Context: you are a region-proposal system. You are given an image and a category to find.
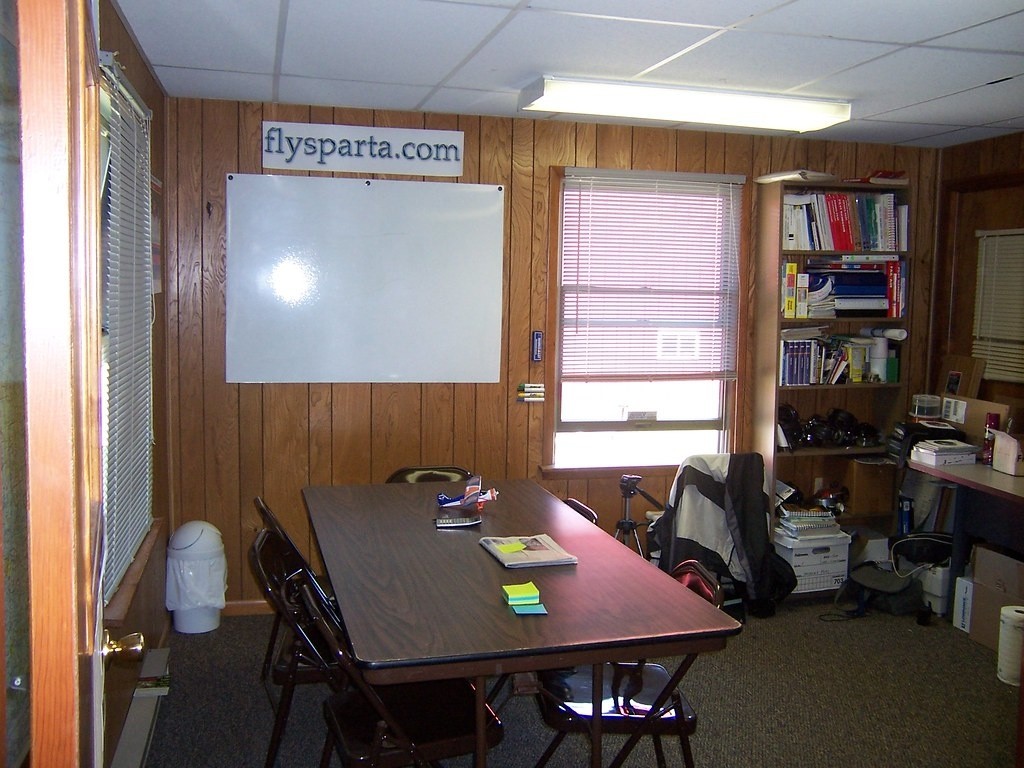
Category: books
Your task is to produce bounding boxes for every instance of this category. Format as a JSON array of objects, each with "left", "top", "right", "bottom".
[
  {"left": 915, "top": 441, "right": 979, "bottom": 467},
  {"left": 781, "top": 191, "right": 907, "bottom": 385},
  {"left": 773, "top": 501, "right": 840, "bottom": 539}
]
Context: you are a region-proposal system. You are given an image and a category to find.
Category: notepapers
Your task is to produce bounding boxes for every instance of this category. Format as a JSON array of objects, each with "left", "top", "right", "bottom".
[{"left": 502, "top": 581, "right": 540, "bottom": 605}]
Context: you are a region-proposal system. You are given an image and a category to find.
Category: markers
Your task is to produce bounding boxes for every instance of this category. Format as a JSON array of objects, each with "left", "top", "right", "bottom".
[{"left": 517, "top": 384, "right": 545, "bottom": 402}]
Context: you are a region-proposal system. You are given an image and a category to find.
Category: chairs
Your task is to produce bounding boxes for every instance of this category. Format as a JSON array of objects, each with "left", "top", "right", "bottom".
[{"left": 246, "top": 466, "right": 724, "bottom": 767}]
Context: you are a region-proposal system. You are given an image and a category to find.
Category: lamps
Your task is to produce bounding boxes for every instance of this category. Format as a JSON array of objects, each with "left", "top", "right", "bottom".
[{"left": 516, "top": 75, "right": 852, "bottom": 134}]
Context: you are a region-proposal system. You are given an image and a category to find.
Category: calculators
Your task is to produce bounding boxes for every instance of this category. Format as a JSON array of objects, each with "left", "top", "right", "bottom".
[{"left": 436, "top": 515, "right": 482, "bottom": 528}]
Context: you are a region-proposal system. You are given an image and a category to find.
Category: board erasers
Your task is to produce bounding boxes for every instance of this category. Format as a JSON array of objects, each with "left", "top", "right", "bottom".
[{"left": 533, "top": 331, "right": 543, "bottom": 361}]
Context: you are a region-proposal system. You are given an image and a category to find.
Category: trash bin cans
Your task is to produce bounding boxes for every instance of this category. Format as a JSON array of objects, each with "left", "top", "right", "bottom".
[{"left": 167, "top": 520, "right": 225, "bottom": 634}]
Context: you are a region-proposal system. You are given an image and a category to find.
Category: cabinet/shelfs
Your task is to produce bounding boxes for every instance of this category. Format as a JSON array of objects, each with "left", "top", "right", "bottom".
[{"left": 755, "top": 181, "right": 915, "bottom": 546}]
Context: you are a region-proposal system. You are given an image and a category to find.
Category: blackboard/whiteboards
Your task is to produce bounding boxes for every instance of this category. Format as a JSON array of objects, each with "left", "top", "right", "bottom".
[{"left": 226, "top": 173, "right": 504, "bottom": 383}]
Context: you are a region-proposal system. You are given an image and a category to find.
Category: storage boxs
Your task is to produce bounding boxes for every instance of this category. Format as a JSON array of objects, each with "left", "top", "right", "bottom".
[
  {"left": 965, "top": 544, "right": 1024, "bottom": 653},
  {"left": 784, "top": 263, "right": 797, "bottom": 318},
  {"left": 950, "top": 578, "right": 975, "bottom": 634},
  {"left": 831, "top": 456, "right": 893, "bottom": 517},
  {"left": 773, "top": 534, "right": 849, "bottom": 594},
  {"left": 934, "top": 354, "right": 1024, "bottom": 476},
  {"left": 841, "top": 343, "right": 862, "bottom": 383},
  {"left": 796, "top": 274, "right": 809, "bottom": 319},
  {"left": 894, "top": 555, "right": 952, "bottom": 619}
]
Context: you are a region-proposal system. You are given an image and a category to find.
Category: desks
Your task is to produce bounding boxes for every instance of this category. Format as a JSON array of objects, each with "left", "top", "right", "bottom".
[
  {"left": 905, "top": 457, "right": 1024, "bottom": 537},
  {"left": 299, "top": 484, "right": 743, "bottom": 767}
]
